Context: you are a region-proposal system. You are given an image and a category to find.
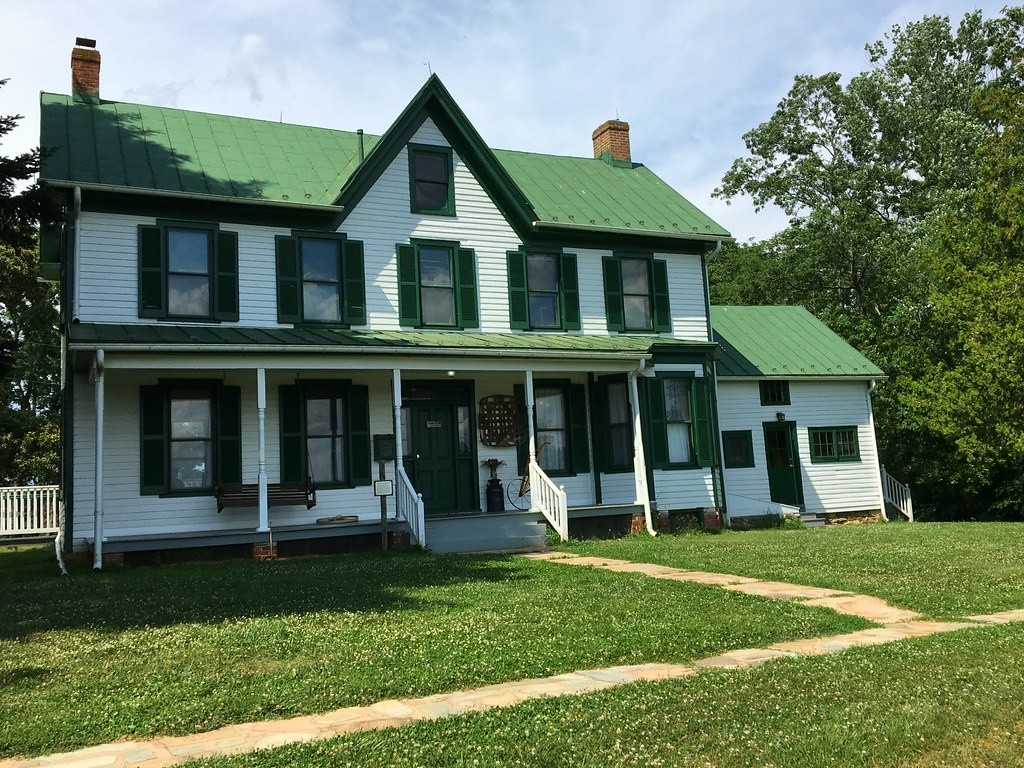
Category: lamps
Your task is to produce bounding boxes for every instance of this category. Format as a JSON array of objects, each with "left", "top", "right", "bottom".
[{"left": 776, "top": 411, "right": 786, "bottom": 421}]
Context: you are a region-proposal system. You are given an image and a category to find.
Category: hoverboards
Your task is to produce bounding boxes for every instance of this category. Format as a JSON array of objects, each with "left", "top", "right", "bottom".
[{"left": 506, "top": 441, "right": 552, "bottom": 511}]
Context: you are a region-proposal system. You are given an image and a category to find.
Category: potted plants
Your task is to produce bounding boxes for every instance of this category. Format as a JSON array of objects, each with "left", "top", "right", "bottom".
[{"left": 482, "top": 457, "right": 508, "bottom": 513}]
